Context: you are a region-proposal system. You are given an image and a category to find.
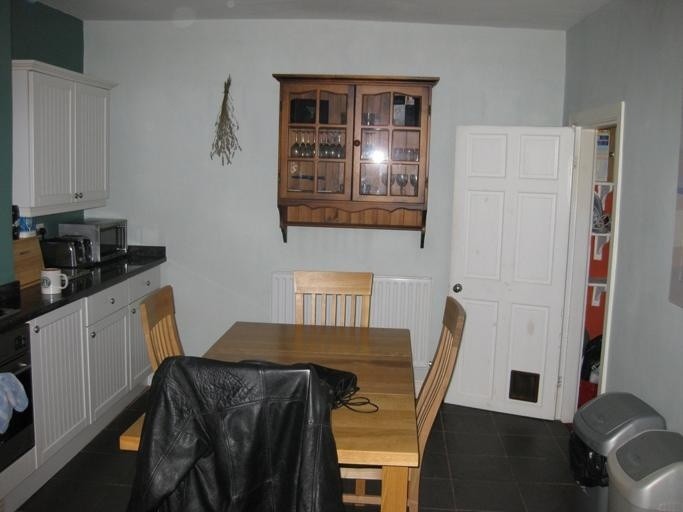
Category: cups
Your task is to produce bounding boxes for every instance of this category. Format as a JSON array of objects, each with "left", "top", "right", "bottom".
[
  {"left": 364, "top": 112, "right": 375, "bottom": 125},
  {"left": 324, "top": 130, "right": 330, "bottom": 158},
  {"left": 330, "top": 130, "right": 337, "bottom": 158},
  {"left": 40, "top": 268, "right": 68, "bottom": 294},
  {"left": 397, "top": 174, "right": 408, "bottom": 196},
  {"left": 311, "top": 130, "right": 315, "bottom": 152},
  {"left": 410, "top": 175, "right": 418, "bottom": 195},
  {"left": 319, "top": 129, "right": 324, "bottom": 158},
  {"left": 383, "top": 173, "right": 395, "bottom": 194},
  {"left": 300, "top": 130, "right": 306, "bottom": 148},
  {"left": 337, "top": 130, "right": 344, "bottom": 158},
  {"left": 303, "top": 130, "right": 313, "bottom": 159},
  {"left": 291, "top": 130, "right": 300, "bottom": 157}
]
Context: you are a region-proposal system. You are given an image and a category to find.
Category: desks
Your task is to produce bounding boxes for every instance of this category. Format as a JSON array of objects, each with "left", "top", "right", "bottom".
[{"left": 120, "top": 321, "right": 419, "bottom": 511}]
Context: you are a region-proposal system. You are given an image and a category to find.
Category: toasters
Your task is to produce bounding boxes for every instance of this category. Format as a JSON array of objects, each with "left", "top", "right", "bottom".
[{"left": 40, "top": 235, "right": 92, "bottom": 270}]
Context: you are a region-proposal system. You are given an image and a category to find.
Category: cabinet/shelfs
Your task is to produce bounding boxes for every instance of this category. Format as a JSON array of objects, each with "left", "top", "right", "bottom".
[
  {"left": 27, "top": 300, "right": 90, "bottom": 469},
  {"left": 11, "top": 59, "right": 119, "bottom": 217},
  {"left": 272, "top": 73, "right": 440, "bottom": 249},
  {"left": 85, "top": 266, "right": 161, "bottom": 424}
]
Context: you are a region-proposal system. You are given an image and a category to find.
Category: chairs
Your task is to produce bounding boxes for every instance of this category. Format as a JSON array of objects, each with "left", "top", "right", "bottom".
[
  {"left": 294, "top": 271, "right": 373, "bottom": 327},
  {"left": 340, "top": 297, "right": 466, "bottom": 512},
  {"left": 140, "top": 285, "right": 185, "bottom": 372}
]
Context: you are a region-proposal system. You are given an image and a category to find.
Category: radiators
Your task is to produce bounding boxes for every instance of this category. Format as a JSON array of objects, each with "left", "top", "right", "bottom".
[{"left": 272, "top": 270, "right": 432, "bottom": 368}]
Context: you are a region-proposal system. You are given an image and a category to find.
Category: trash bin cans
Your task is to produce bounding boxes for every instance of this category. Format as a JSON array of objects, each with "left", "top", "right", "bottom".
[
  {"left": 572, "top": 391, "right": 667, "bottom": 511},
  {"left": 604, "top": 427, "right": 683, "bottom": 512}
]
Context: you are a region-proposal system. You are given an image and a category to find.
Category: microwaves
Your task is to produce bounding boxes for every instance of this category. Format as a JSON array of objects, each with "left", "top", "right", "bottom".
[{"left": 59, "top": 217, "right": 127, "bottom": 262}]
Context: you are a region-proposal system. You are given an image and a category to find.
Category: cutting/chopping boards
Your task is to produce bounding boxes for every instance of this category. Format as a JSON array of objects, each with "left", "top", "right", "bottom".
[{"left": 13, "top": 236, "right": 44, "bottom": 289}]
